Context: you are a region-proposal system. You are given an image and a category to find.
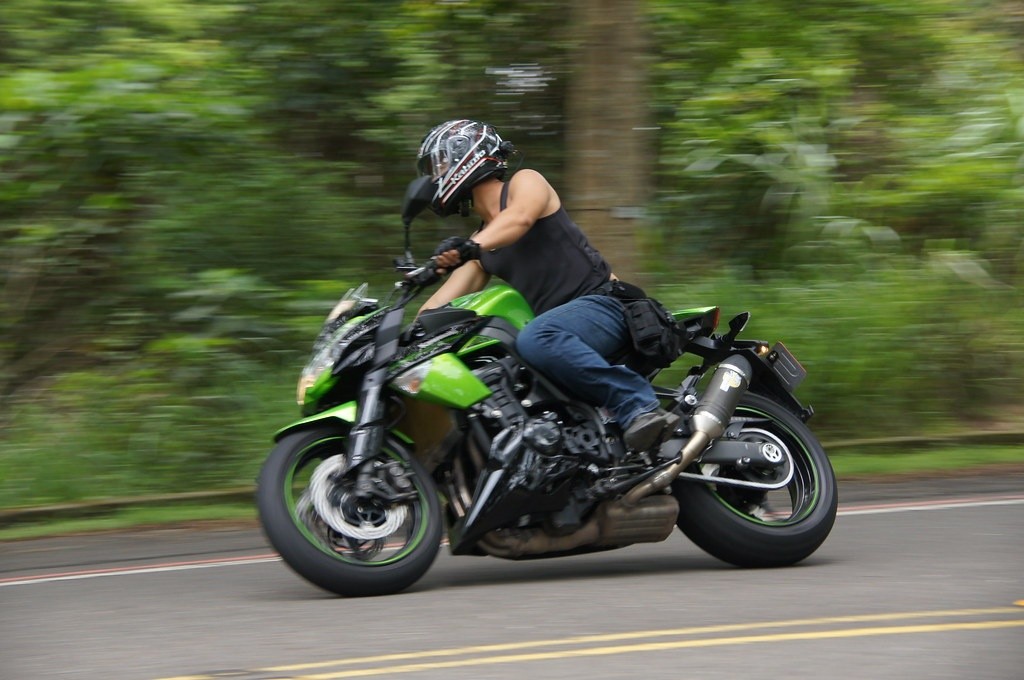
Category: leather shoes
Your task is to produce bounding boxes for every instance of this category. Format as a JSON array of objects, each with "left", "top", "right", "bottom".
[{"left": 625, "top": 407, "right": 681, "bottom": 451}]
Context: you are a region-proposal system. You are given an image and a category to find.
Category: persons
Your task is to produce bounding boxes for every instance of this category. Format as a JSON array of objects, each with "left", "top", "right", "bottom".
[{"left": 414, "top": 119, "right": 679, "bottom": 450}]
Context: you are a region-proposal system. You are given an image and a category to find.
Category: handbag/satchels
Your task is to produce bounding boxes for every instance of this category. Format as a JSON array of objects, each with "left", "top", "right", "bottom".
[{"left": 626, "top": 297, "right": 678, "bottom": 360}]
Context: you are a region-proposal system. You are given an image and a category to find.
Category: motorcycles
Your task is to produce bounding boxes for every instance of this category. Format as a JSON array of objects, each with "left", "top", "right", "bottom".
[{"left": 253, "top": 176, "right": 839, "bottom": 599}]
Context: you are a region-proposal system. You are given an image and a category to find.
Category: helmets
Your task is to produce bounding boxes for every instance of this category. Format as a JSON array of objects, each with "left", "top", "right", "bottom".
[{"left": 417, "top": 118, "right": 510, "bottom": 216}]
